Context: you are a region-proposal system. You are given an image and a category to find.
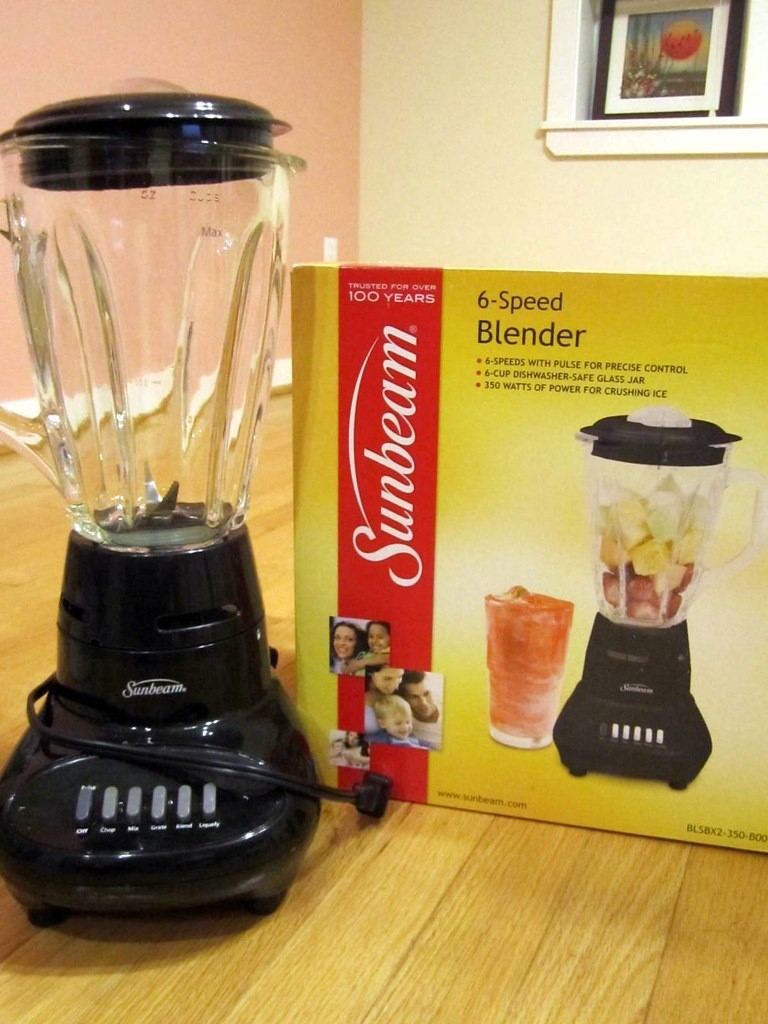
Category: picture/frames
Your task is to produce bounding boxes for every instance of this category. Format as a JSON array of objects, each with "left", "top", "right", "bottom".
[{"left": 592, "top": 0, "right": 744, "bottom": 119}]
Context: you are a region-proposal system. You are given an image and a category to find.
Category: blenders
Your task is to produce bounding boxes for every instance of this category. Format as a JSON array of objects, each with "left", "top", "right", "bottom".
[
  {"left": 548, "top": 405, "right": 747, "bottom": 793},
  {"left": 0, "top": 69, "right": 323, "bottom": 926}
]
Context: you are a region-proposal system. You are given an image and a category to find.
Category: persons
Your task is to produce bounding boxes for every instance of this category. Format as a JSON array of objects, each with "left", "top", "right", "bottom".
[
  {"left": 398, "top": 668, "right": 444, "bottom": 748},
  {"left": 328, "top": 621, "right": 365, "bottom": 676},
  {"left": 365, "top": 695, "right": 436, "bottom": 749},
  {"left": 339, "top": 621, "right": 391, "bottom": 677},
  {"left": 329, "top": 729, "right": 372, "bottom": 771},
  {"left": 365, "top": 662, "right": 404, "bottom": 734}
]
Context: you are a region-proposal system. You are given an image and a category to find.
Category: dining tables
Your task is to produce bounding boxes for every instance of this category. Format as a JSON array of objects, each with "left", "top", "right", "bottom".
[{"left": 0, "top": 386, "right": 768, "bottom": 1024}]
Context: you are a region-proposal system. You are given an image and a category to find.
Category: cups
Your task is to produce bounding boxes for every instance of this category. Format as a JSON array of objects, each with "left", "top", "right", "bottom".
[{"left": 482, "top": 594, "right": 573, "bottom": 753}]
